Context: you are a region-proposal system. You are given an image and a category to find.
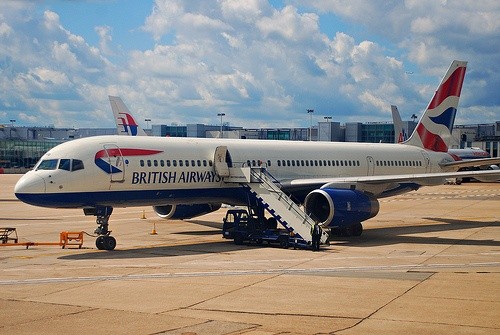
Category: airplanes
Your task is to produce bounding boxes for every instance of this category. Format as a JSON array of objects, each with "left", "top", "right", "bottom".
[{"left": 14, "top": 59, "right": 500, "bottom": 252}]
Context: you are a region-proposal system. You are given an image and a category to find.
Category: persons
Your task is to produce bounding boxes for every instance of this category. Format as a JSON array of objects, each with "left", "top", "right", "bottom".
[{"left": 310, "top": 221, "right": 322, "bottom": 252}]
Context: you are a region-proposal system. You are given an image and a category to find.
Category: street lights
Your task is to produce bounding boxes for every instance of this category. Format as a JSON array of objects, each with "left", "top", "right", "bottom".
[
  {"left": 411, "top": 114, "right": 418, "bottom": 122},
  {"left": 307, "top": 108, "right": 314, "bottom": 141},
  {"left": 217, "top": 113, "right": 225, "bottom": 133},
  {"left": 324, "top": 116, "right": 333, "bottom": 122},
  {"left": 145, "top": 118, "right": 151, "bottom": 128},
  {"left": 10, "top": 119, "right": 16, "bottom": 125}
]
